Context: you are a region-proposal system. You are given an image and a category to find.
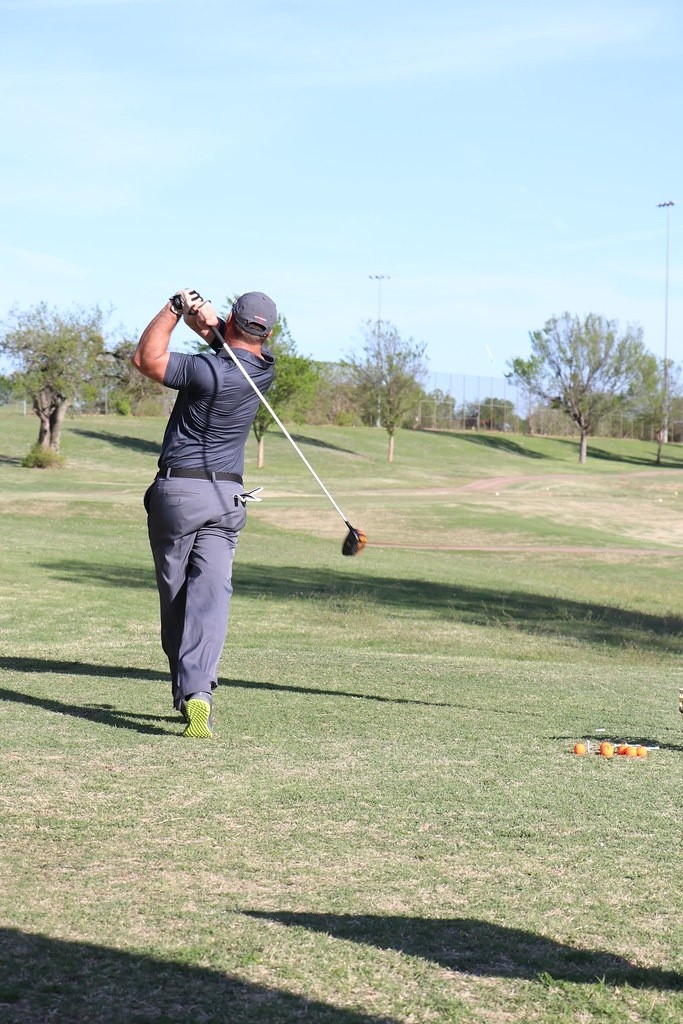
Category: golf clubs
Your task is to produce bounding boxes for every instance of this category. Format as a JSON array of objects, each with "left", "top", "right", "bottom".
[{"left": 185, "top": 285, "right": 368, "bottom": 557}]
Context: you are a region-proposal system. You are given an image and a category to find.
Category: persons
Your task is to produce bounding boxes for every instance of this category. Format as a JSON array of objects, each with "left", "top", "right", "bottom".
[{"left": 132, "top": 287, "right": 278, "bottom": 738}]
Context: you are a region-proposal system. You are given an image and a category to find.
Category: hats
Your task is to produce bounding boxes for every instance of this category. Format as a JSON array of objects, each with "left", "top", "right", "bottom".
[{"left": 232, "top": 292, "right": 277, "bottom": 337}]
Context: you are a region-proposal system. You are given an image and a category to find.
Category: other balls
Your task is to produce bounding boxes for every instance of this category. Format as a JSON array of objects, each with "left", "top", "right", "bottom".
[
  {"left": 573, "top": 743, "right": 586, "bottom": 756},
  {"left": 600, "top": 741, "right": 647, "bottom": 758}
]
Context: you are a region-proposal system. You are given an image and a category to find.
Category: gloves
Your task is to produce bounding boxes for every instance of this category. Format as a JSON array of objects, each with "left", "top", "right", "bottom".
[
  {"left": 233, "top": 486, "right": 264, "bottom": 502},
  {"left": 169, "top": 288, "right": 211, "bottom": 317}
]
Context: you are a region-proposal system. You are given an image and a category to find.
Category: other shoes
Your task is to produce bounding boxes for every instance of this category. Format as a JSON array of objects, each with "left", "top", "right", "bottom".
[{"left": 178, "top": 692, "right": 213, "bottom": 738}]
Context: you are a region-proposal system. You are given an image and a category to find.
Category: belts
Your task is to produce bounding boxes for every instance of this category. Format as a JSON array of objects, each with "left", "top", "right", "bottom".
[{"left": 158, "top": 469, "right": 243, "bottom": 485}]
